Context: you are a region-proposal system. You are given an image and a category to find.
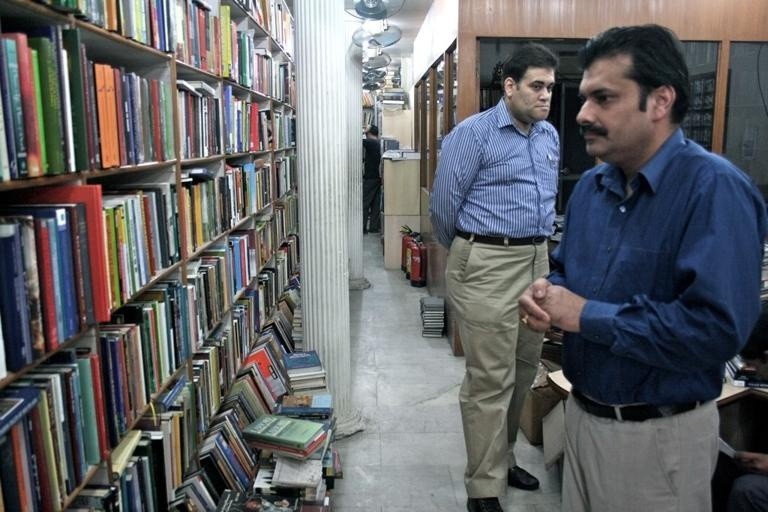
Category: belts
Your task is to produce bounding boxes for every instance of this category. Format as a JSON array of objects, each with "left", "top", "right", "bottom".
[
  {"left": 456, "top": 229, "right": 546, "bottom": 246},
  {"left": 572, "top": 387, "right": 705, "bottom": 422}
]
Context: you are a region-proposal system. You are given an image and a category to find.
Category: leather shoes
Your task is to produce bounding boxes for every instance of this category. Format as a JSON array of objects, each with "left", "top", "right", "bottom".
[
  {"left": 467, "top": 496, "right": 503, "bottom": 512},
  {"left": 508, "top": 465, "right": 539, "bottom": 491}
]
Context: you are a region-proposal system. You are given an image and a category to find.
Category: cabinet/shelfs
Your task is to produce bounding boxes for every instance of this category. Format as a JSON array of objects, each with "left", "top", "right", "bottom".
[
  {"left": 0, "top": 0, "right": 299, "bottom": 511},
  {"left": 378, "top": 109, "right": 412, "bottom": 156},
  {"left": 383, "top": 158, "right": 421, "bottom": 269},
  {"left": 375, "top": 90, "right": 404, "bottom": 127}
]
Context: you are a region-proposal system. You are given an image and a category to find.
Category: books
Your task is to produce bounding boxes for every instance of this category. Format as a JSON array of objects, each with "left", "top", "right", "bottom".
[
  {"left": 0, "top": 153, "right": 342, "bottom": 421},
  {"left": 419, "top": 296, "right": 445, "bottom": 337},
  {"left": 2, "top": 23, "right": 297, "bottom": 180},
  {"left": 384, "top": 140, "right": 421, "bottom": 161},
  {"left": 48, "top": 1, "right": 297, "bottom": 112},
  {"left": 0, "top": 186, "right": 343, "bottom": 509}
]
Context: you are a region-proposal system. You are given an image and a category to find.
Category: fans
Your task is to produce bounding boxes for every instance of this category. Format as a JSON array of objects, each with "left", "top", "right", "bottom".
[
  {"left": 351, "top": 21, "right": 402, "bottom": 49},
  {"left": 353, "top": 51, "right": 391, "bottom": 90},
  {"left": 344, "top": 0, "right": 406, "bottom": 21}
]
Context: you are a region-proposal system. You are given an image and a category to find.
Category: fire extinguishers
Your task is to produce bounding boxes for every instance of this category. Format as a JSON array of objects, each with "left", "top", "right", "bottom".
[{"left": 400, "top": 225, "right": 427, "bottom": 287}]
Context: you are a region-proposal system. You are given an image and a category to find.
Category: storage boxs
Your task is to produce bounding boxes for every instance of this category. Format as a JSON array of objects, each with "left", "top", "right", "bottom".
[
  {"left": 542, "top": 401, "right": 741, "bottom": 493},
  {"left": 520, "top": 386, "right": 566, "bottom": 447}
]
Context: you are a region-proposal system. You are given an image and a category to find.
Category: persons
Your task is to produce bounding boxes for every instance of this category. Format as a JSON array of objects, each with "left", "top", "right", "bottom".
[
  {"left": 362, "top": 125, "right": 382, "bottom": 233},
  {"left": 515, "top": 22, "right": 767, "bottom": 512},
  {"left": 430, "top": 42, "right": 562, "bottom": 512}
]
